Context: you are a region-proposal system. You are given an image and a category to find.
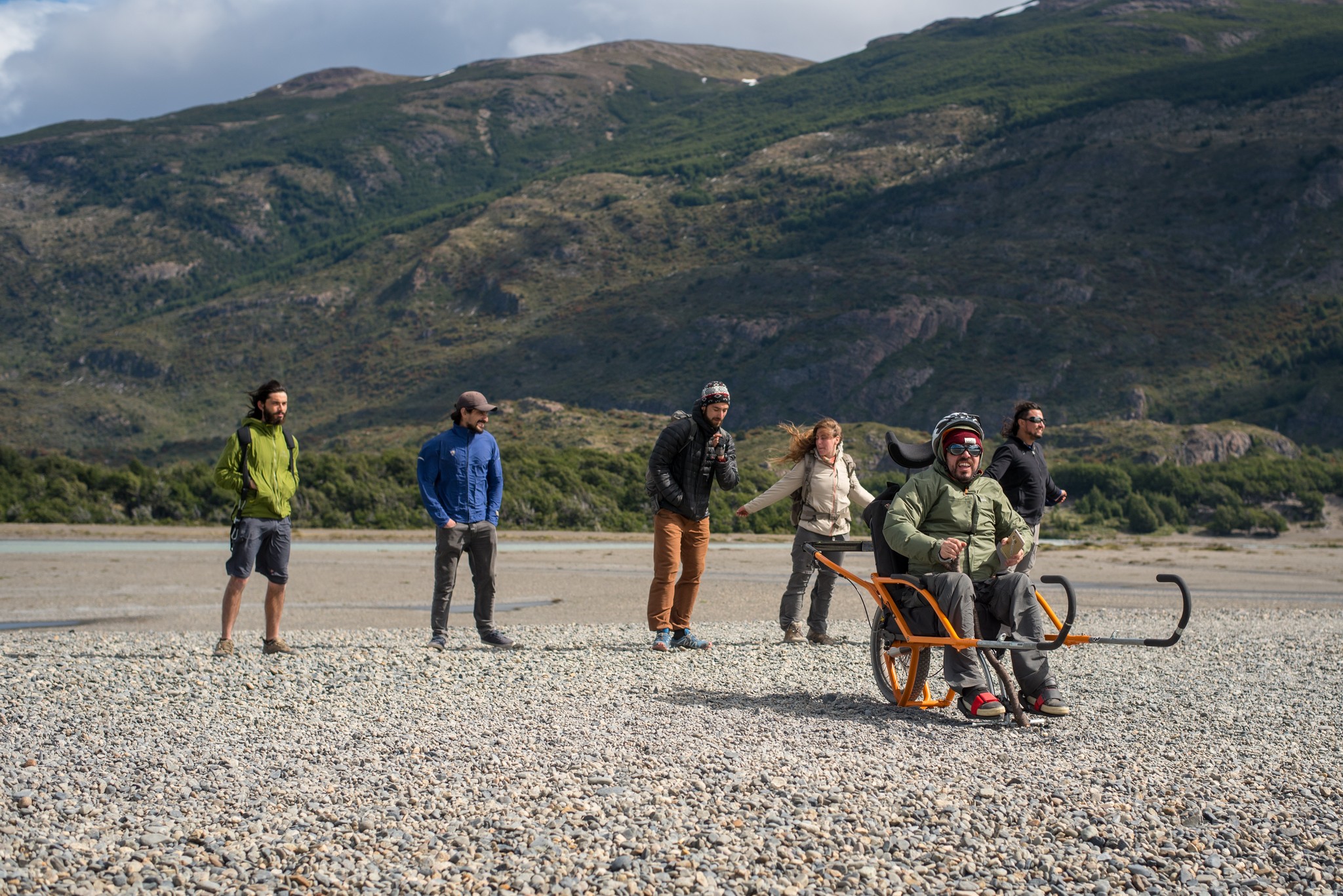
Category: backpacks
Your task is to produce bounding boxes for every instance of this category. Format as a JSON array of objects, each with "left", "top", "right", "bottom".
[
  {"left": 789, "top": 449, "right": 856, "bottom": 528},
  {"left": 643, "top": 410, "right": 698, "bottom": 513}
]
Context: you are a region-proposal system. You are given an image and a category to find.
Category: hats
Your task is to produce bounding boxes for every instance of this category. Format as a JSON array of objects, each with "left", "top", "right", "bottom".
[
  {"left": 456, "top": 391, "right": 498, "bottom": 412},
  {"left": 701, "top": 381, "right": 730, "bottom": 408}
]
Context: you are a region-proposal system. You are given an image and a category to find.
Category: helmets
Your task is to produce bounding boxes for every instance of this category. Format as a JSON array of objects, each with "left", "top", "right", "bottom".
[{"left": 931, "top": 412, "right": 985, "bottom": 457}]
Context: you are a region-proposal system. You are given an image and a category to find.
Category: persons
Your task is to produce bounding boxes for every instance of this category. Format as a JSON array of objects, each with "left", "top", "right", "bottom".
[
  {"left": 214, "top": 380, "right": 303, "bottom": 656},
  {"left": 416, "top": 390, "right": 517, "bottom": 653},
  {"left": 880, "top": 412, "right": 1071, "bottom": 717},
  {"left": 734, "top": 418, "right": 877, "bottom": 645},
  {"left": 645, "top": 380, "right": 739, "bottom": 651},
  {"left": 982, "top": 401, "right": 1067, "bottom": 577}
]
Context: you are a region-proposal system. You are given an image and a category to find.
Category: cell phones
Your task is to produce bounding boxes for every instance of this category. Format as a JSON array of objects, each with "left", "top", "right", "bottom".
[{"left": 1000, "top": 529, "right": 1025, "bottom": 560}]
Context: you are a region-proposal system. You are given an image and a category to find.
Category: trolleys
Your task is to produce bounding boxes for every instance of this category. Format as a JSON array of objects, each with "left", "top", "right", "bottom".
[{"left": 798, "top": 427, "right": 1194, "bottom": 710}]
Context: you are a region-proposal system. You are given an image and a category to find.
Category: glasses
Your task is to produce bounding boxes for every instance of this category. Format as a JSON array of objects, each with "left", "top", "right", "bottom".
[
  {"left": 947, "top": 444, "right": 982, "bottom": 457},
  {"left": 1026, "top": 416, "right": 1047, "bottom": 425}
]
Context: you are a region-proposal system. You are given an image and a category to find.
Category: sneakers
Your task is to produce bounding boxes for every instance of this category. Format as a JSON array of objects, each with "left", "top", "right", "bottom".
[
  {"left": 807, "top": 628, "right": 837, "bottom": 646},
  {"left": 214, "top": 637, "right": 235, "bottom": 656},
  {"left": 652, "top": 628, "right": 671, "bottom": 651},
  {"left": 1028, "top": 676, "right": 1069, "bottom": 714},
  {"left": 671, "top": 629, "right": 709, "bottom": 650},
  {"left": 427, "top": 635, "right": 446, "bottom": 651},
  {"left": 262, "top": 637, "right": 301, "bottom": 656},
  {"left": 481, "top": 629, "right": 515, "bottom": 647},
  {"left": 962, "top": 683, "right": 1006, "bottom": 716},
  {"left": 783, "top": 621, "right": 809, "bottom": 644}
]
